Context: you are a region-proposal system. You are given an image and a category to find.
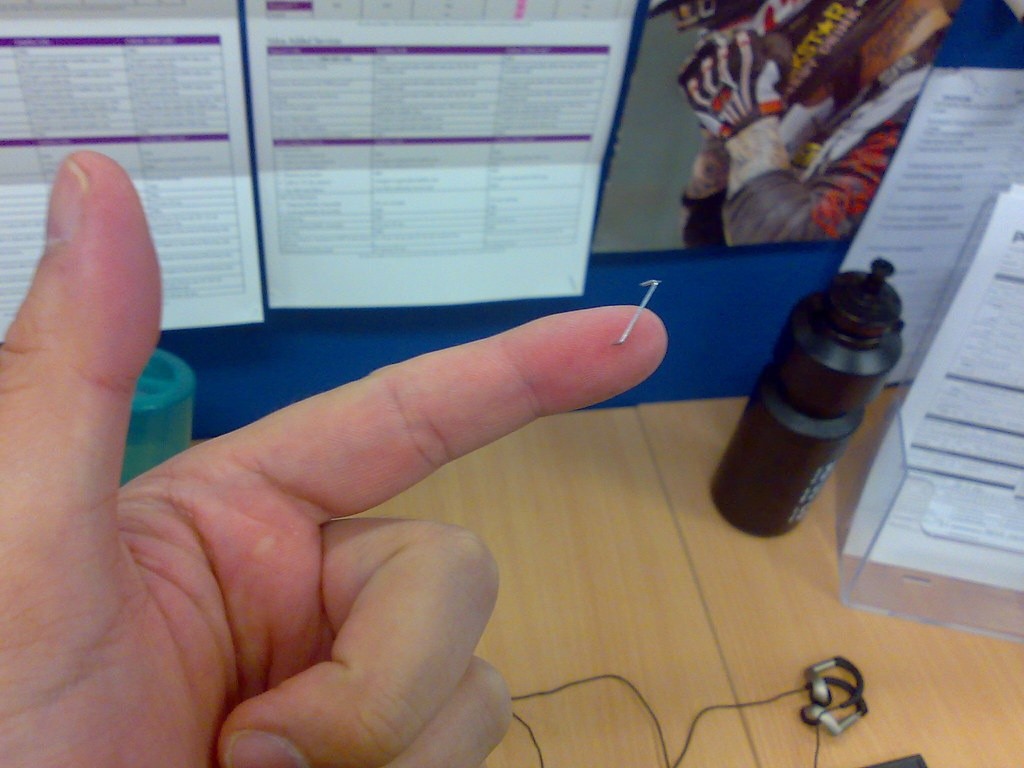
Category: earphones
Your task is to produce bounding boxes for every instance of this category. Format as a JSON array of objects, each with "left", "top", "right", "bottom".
[{"left": 800, "top": 657, "right": 869, "bottom": 736}]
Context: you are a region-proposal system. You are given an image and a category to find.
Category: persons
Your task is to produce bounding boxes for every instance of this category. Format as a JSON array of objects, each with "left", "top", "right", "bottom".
[
  {"left": 649, "top": 0, "right": 956, "bottom": 246},
  {"left": 1, "top": 151, "right": 668, "bottom": 768}
]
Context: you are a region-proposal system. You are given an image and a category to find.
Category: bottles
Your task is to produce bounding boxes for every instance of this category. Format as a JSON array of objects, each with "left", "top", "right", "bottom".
[{"left": 710, "top": 255, "right": 906, "bottom": 540}]
